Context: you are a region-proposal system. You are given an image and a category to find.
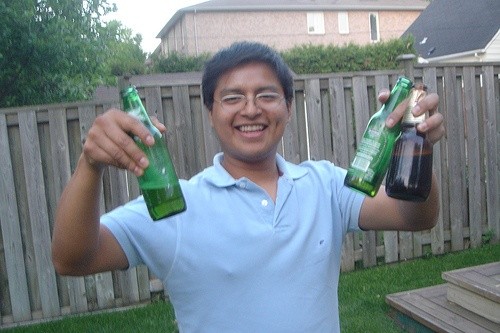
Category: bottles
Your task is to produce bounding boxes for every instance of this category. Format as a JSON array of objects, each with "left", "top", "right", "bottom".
[
  {"left": 343, "top": 76, "right": 413, "bottom": 198},
  {"left": 385, "top": 84, "right": 434, "bottom": 201},
  {"left": 119, "top": 84, "right": 188, "bottom": 222}
]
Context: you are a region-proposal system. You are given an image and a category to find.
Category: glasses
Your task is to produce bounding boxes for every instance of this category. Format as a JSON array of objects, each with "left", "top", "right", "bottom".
[{"left": 213, "top": 92, "right": 285, "bottom": 110}]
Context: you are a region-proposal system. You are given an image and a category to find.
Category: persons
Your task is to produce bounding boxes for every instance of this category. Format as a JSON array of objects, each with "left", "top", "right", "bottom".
[{"left": 52, "top": 41, "right": 445, "bottom": 333}]
